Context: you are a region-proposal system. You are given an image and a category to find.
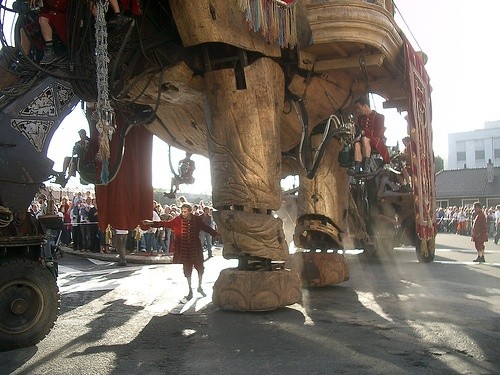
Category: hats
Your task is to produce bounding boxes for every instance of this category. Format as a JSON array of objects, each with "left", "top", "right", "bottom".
[
  {"left": 182, "top": 204, "right": 191, "bottom": 211},
  {"left": 78, "top": 129, "right": 87, "bottom": 133}
]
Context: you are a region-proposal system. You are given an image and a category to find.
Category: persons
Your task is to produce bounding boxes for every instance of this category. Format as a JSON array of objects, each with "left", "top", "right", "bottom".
[
  {"left": 11, "top": 0, "right": 143, "bottom": 67},
  {"left": 471, "top": 202, "right": 488, "bottom": 262},
  {"left": 493, "top": 205, "right": 500, "bottom": 244},
  {"left": 163, "top": 150, "right": 196, "bottom": 199},
  {"left": 55, "top": 129, "right": 90, "bottom": 188},
  {"left": 126, "top": 195, "right": 219, "bottom": 257},
  {"left": 395, "top": 137, "right": 418, "bottom": 193},
  {"left": 343, "top": 97, "right": 391, "bottom": 176},
  {"left": 140, "top": 202, "right": 222, "bottom": 301},
  {"left": 434, "top": 204, "right": 500, "bottom": 238},
  {"left": 27, "top": 191, "right": 115, "bottom": 255}
]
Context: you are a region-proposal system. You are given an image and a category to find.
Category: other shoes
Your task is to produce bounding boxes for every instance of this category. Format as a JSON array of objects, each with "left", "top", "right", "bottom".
[
  {"left": 401, "top": 184, "right": 413, "bottom": 191},
  {"left": 476, "top": 256, "right": 485, "bottom": 262},
  {"left": 473, "top": 258, "right": 479, "bottom": 262},
  {"left": 348, "top": 161, "right": 362, "bottom": 175},
  {"left": 362, "top": 157, "right": 370, "bottom": 174}
]
